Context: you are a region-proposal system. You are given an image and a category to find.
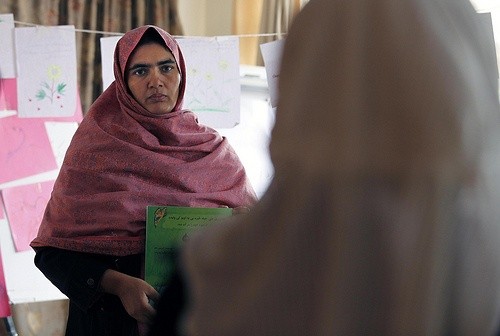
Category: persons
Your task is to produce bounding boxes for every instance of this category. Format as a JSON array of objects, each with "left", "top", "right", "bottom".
[
  {"left": 145, "top": 3, "right": 498, "bottom": 335},
  {"left": 28, "top": 25, "right": 258, "bottom": 336}
]
